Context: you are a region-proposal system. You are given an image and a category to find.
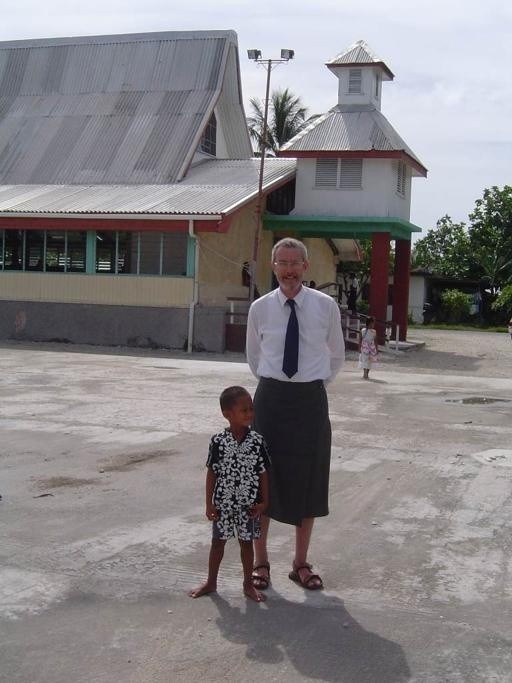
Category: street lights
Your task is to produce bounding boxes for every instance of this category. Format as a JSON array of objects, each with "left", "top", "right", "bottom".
[{"left": 247, "top": 47, "right": 295, "bottom": 301}]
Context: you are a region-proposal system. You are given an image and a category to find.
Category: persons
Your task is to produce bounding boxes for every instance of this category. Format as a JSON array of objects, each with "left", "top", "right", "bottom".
[
  {"left": 186, "top": 383, "right": 273, "bottom": 603},
  {"left": 243, "top": 235, "right": 346, "bottom": 590},
  {"left": 348, "top": 271, "right": 361, "bottom": 313},
  {"left": 356, "top": 315, "right": 379, "bottom": 378}
]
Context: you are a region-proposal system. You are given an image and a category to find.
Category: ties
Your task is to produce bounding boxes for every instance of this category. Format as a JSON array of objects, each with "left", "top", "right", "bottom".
[{"left": 283, "top": 299, "right": 299, "bottom": 378}]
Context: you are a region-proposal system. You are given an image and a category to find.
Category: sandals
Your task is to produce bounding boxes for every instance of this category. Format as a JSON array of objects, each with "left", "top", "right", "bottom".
[
  {"left": 251, "top": 561, "right": 270, "bottom": 589},
  {"left": 289, "top": 560, "right": 322, "bottom": 590}
]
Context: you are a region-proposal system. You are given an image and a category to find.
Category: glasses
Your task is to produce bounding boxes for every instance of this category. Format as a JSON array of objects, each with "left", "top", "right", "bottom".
[{"left": 274, "top": 262, "right": 305, "bottom": 270}]
